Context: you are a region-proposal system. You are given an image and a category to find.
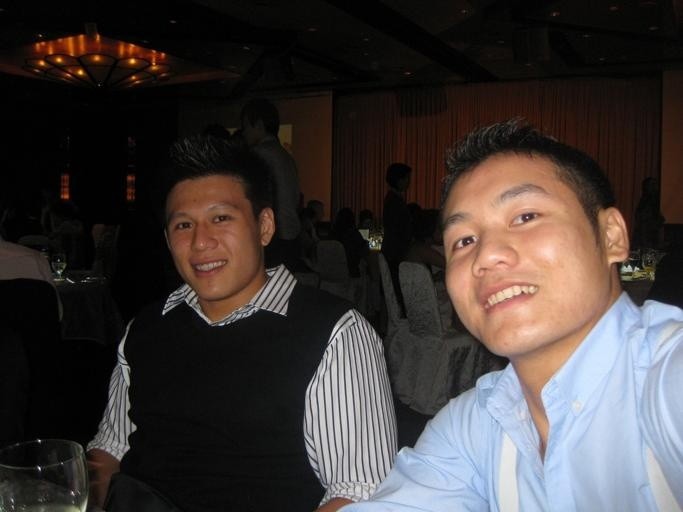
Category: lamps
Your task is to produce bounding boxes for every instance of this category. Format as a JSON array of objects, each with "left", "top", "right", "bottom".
[{"left": 17, "top": 29, "right": 174, "bottom": 101}]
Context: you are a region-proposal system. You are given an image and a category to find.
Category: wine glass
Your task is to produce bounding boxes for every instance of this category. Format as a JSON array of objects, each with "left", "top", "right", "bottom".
[
  {"left": 36, "top": 246, "right": 68, "bottom": 282},
  {"left": 620, "top": 247, "right": 667, "bottom": 281}
]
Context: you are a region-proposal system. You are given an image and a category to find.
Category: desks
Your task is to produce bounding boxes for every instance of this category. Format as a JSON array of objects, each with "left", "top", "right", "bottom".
[
  {"left": 49, "top": 266, "right": 128, "bottom": 341},
  {"left": 618, "top": 265, "right": 656, "bottom": 308}
]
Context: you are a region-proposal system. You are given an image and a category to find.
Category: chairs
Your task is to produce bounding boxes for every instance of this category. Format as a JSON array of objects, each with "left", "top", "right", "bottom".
[
  {"left": 1, "top": 323, "right": 35, "bottom": 464},
  {"left": 291, "top": 233, "right": 506, "bottom": 416}
]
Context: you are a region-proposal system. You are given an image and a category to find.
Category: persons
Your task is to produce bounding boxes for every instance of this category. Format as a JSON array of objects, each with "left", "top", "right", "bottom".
[
  {"left": 334, "top": 117, "right": 683, "bottom": 512},
  {"left": 84, "top": 166, "right": 397, "bottom": 512},
  {"left": 644, "top": 251, "right": 683, "bottom": 309},
  {"left": 630, "top": 177, "right": 664, "bottom": 252},
  {"left": 241, "top": 98, "right": 445, "bottom": 287},
  {"left": 1, "top": 239, "right": 63, "bottom": 322}
]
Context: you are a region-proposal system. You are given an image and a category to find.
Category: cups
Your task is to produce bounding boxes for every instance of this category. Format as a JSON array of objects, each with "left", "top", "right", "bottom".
[{"left": 0, "top": 439, "right": 89, "bottom": 512}]
